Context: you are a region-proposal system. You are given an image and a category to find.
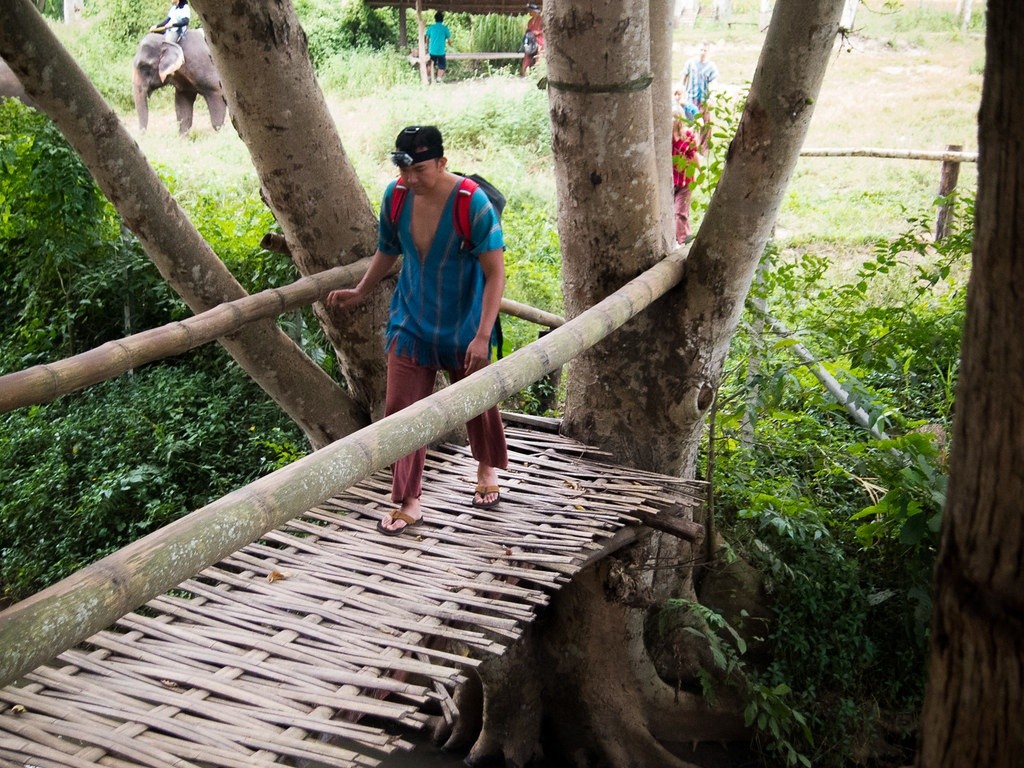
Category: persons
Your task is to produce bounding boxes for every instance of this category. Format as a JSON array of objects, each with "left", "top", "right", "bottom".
[
  {"left": 672, "top": 40, "right": 718, "bottom": 244},
  {"left": 523, "top": 3, "right": 545, "bottom": 63},
  {"left": 149, "top": 0, "right": 191, "bottom": 77},
  {"left": 425, "top": 11, "right": 452, "bottom": 85},
  {"left": 326, "top": 125, "right": 505, "bottom": 536}
]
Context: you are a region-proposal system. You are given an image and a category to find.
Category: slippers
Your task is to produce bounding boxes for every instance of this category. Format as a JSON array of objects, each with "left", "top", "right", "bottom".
[
  {"left": 376, "top": 510, "right": 424, "bottom": 536},
  {"left": 472, "top": 485, "right": 500, "bottom": 507}
]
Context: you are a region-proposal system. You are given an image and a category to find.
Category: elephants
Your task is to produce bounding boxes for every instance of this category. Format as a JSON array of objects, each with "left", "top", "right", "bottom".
[{"left": 132, "top": 28, "right": 227, "bottom": 140}]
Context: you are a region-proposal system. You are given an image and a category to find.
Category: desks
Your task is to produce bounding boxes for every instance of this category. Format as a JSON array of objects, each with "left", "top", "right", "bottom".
[{"left": 408, "top": 52, "right": 532, "bottom": 84}]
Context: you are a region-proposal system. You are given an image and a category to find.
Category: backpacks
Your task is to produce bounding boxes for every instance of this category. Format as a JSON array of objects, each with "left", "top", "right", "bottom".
[{"left": 390, "top": 171, "right": 506, "bottom": 250}]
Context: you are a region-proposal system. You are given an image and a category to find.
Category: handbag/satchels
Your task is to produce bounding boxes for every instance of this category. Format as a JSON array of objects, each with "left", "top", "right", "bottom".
[{"left": 524, "top": 30, "right": 537, "bottom": 55}]
